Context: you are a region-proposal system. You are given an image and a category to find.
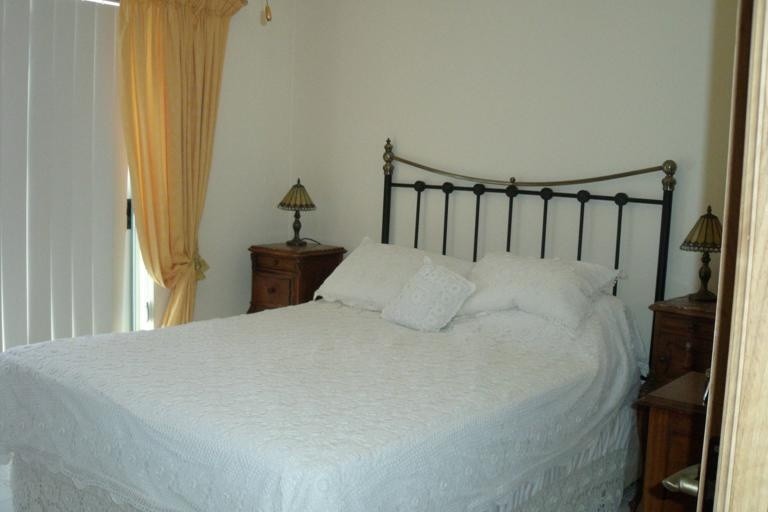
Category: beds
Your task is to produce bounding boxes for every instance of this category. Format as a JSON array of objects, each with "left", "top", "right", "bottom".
[{"left": 0, "top": 140, "right": 676, "bottom": 512}]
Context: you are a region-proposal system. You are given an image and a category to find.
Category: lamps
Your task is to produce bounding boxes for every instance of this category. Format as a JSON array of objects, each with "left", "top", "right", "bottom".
[
  {"left": 677, "top": 204, "right": 731, "bottom": 305},
  {"left": 277, "top": 176, "right": 316, "bottom": 247}
]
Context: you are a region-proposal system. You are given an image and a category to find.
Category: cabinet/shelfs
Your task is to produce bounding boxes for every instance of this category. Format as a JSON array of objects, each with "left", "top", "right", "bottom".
[
  {"left": 630, "top": 291, "right": 716, "bottom": 393},
  {"left": 246, "top": 242, "right": 347, "bottom": 314}
]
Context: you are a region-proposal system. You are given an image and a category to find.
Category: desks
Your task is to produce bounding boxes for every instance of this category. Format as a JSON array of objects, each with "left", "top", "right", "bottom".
[{"left": 631, "top": 366, "right": 709, "bottom": 511}]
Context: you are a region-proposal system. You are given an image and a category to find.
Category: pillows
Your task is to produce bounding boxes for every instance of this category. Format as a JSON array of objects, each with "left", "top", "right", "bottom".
[
  {"left": 378, "top": 254, "right": 477, "bottom": 332},
  {"left": 455, "top": 247, "right": 624, "bottom": 333},
  {"left": 313, "top": 237, "right": 473, "bottom": 313}
]
